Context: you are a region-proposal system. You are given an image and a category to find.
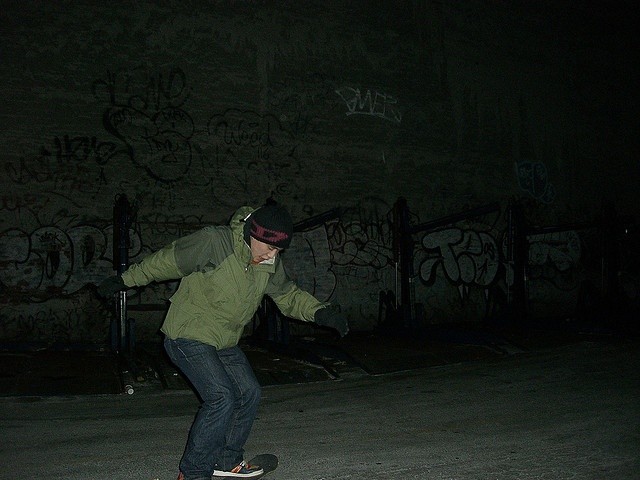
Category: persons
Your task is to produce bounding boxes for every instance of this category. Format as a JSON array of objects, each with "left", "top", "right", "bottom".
[{"left": 98, "top": 204, "right": 350, "bottom": 478}]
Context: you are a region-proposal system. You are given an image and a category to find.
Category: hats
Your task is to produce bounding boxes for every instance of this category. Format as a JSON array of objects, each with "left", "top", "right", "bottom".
[{"left": 252, "top": 206, "right": 292, "bottom": 248}]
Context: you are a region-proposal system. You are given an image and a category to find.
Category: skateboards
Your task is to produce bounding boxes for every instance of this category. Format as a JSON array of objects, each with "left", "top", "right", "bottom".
[{"left": 216, "top": 453, "right": 279, "bottom": 479}]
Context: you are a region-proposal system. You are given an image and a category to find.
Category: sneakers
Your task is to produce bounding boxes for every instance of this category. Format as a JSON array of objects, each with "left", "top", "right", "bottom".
[{"left": 212, "top": 460, "right": 264, "bottom": 478}]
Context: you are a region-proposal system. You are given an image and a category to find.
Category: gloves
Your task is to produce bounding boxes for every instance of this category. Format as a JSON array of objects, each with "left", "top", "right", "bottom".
[
  {"left": 315, "top": 304, "right": 349, "bottom": 336},
  {"left": 100, "top": 276, "right": 125, "bottom": 294}
]
